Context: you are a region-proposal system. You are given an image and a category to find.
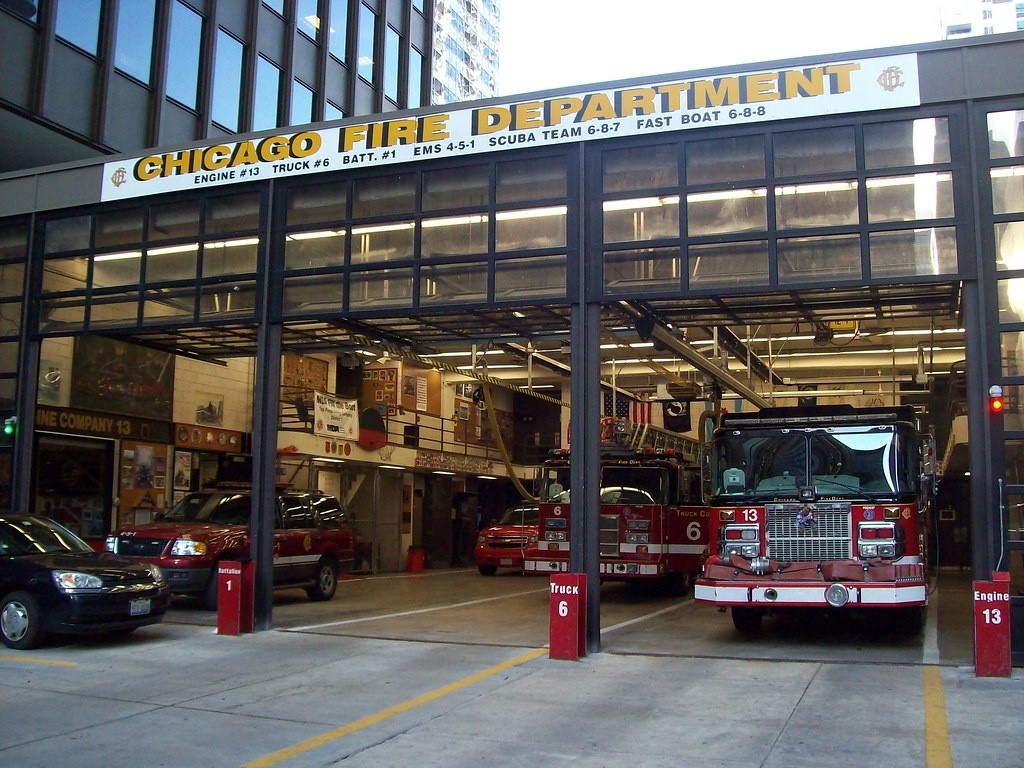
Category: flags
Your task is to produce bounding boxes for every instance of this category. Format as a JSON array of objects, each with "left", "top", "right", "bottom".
[{"left": 604, "top": 391, "right": 652, "bottom": 425}]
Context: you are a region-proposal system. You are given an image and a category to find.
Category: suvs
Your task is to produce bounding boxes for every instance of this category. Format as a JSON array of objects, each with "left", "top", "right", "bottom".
[{"left": 102, "top": 482, "right": 351, "bottom": 604}]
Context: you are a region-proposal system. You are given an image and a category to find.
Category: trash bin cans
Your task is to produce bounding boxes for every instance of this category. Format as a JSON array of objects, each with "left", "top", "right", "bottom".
[{"left": 406, "top": 543, "right": 426, "bottom": 573}]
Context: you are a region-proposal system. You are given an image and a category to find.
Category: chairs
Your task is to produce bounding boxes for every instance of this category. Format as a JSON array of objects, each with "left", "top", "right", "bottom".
[{"left": 295, "top": 398, "right": 314, "bottom": 426}]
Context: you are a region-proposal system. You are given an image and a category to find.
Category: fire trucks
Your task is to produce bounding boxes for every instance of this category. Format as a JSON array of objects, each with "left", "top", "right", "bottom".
[
  {"left": 522, "top": 419, "right": 707, "bottom": 602},
  {"left": 694, "top": 404, "right": 942, "bottom": 635}
]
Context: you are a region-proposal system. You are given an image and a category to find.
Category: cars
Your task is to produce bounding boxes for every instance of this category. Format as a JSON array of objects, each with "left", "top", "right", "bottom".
[
  {"left": 476, "top": 502, "right": 538, "bottom": 578},
  {"left": 0, "top": 513, "right": 171, "bottom": 652}
]
